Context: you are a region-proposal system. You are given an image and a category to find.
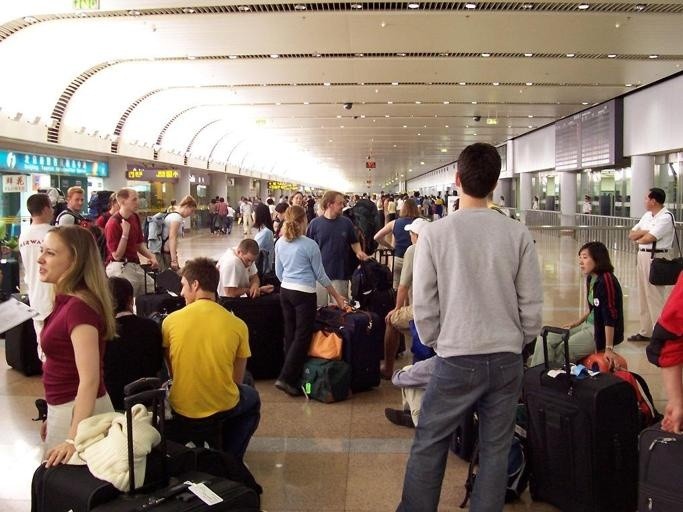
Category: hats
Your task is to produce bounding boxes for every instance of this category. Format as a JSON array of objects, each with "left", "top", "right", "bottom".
[{"left": 404, "top": 217, "right": 431, "bottom": 236}]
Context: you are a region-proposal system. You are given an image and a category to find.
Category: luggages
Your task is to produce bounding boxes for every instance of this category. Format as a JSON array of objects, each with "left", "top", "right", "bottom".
[
  {"left": 5, "top": 292, "right": 44, "bottom": 377},
  {"left": 351, "top": 249, "right": 405, "bottom": 354},
  {"left": 523, "top": 326, "right": 682, "bottom": 511},
  {"left": 32, "top": 377, "right": 260, "bottom": 512},
  {"left": 136, "top": 264, "right": 186, "bottom": 320},
  {"left": 1, "top": 247, "right": 20, "bottom": 301}
]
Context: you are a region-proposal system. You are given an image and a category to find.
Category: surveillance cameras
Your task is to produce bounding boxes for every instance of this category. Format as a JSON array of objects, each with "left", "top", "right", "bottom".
[
  {"left": 473, "top": 116, "right": 481, "bottom": 121},
  {"left": 343, "top": 103, "right": 352, "bottom": 110}
]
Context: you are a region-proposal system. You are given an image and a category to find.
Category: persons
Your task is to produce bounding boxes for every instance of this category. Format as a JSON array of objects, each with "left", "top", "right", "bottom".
[
  {"left": 531, "top": 240, "right": 625, "bottom": 372},
  {"left": 15, "top": 188, "right": 218, "bottom": 368},
  {"left": 215, "top": 190, "right": 462, "bottom": 427},
  {"left": 99, "top": 276, "right": 165, "bottom": 411},
  {"left": 35, "top": 226, "right": 117, "bottom": 467},
  {"left": 532, "top": 196, "right": 539, "bottom": 210},
  {"left": 583, "top": 195, "right": 592, "bottom": 214},
  {"left": 646, "top": 269, "right": 681, "bottom": 435},
  {"left": 396, "top": 142, "right": 542, "bottom": 511},
  {"left": 627, "top": 186, "right": 676, "bottom": 341},
  {"left": 160, "top": 257, "right": 261, "bottom": 460}
]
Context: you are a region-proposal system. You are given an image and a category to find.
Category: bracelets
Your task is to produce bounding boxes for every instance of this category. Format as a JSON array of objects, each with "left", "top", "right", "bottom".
[
  {"left": 63, "top": 437, "right": 75, "bottom": 445},
  {"left": 605, "top": 347, "right": 613, "bottom": 351}
]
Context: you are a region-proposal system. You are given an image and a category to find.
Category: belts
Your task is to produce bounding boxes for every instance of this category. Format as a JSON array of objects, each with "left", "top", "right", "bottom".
[{"left": 641, "top": 248, "right": 667, "bottom": 253}]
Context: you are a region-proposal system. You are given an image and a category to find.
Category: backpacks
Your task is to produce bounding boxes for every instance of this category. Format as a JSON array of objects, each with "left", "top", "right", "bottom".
[
  {"left": 58, "top": 212, "right": 106, "bottom": 262},
  {"left": 146, "top": 211, "right": 180, "bottom": 255},
  {"left": 468, "top": 422, "right": 528, "bottom": 503}
]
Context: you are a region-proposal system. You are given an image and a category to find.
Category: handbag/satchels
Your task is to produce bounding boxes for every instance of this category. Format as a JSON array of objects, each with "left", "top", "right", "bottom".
[
  {"left": 303, "top": 305, "right": 381, "bottom": 404},
  {"left": 649, "top": 257, "right": 681, "bottom": 285}
]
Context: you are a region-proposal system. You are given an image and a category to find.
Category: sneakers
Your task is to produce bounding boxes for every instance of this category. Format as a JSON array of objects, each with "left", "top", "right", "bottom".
[
  {"left": 384, "top": 407, "right": 415, "bottom": 427},
  {"left": 273, "top": 375, "right": 299, "bottom": 394}
]
